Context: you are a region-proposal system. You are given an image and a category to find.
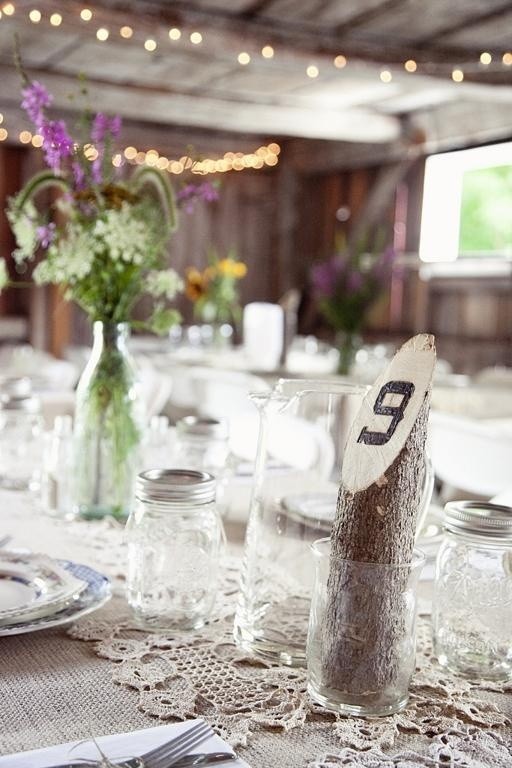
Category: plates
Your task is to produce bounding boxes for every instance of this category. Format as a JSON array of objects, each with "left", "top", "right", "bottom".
[
  {"left": 0, "top": 552, "right": 90, "bottom": 628},
  {"left": 0, "top": 549, "right": 113, "bottom": 640}
]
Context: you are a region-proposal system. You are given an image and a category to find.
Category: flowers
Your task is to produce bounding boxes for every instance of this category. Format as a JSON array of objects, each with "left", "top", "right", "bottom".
[
  {"left": 185, "top": 256, "right": 246, "bottom": 325},
  {"left": 312, "top": 229, "right": 401, "bottom": 330},
  {"left": 0, "top": 79, "right": 220, "bottom": 505}
]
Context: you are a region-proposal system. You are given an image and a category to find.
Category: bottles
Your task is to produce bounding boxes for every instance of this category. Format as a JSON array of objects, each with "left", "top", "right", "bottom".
[
  {"left": 431, "top": 499, "right": 512, "bottom": 682},
  {"left": 0, "top": 370, "right": 78, "bottom": 520},
  {"left": 166, "top": 415, "right": 233, "bottom": 523},
  {"left": 121, "top": 465, "right": 231, "bottom": 631}
]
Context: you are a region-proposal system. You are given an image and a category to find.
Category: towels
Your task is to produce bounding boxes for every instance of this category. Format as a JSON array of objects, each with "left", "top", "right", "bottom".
[{"left": 0, "top": 718, "right": 251, "bottom": 768}]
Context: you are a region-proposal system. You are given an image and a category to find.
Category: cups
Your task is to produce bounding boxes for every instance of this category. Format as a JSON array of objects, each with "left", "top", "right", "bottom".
[
  {"left": 304, "top": 535, "right": 426, "bottom": 720},
  {"left": 230, "top": 372, "right": 439, "bottom": 673}
]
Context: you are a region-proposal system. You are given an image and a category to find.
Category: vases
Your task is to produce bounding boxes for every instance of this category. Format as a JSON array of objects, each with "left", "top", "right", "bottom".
[
  {"left": 73, "top": 321, "right": 146, "bottom": 525},
  {"left": 339, "top": 329, "right": 359, "bottom": 371}
]
